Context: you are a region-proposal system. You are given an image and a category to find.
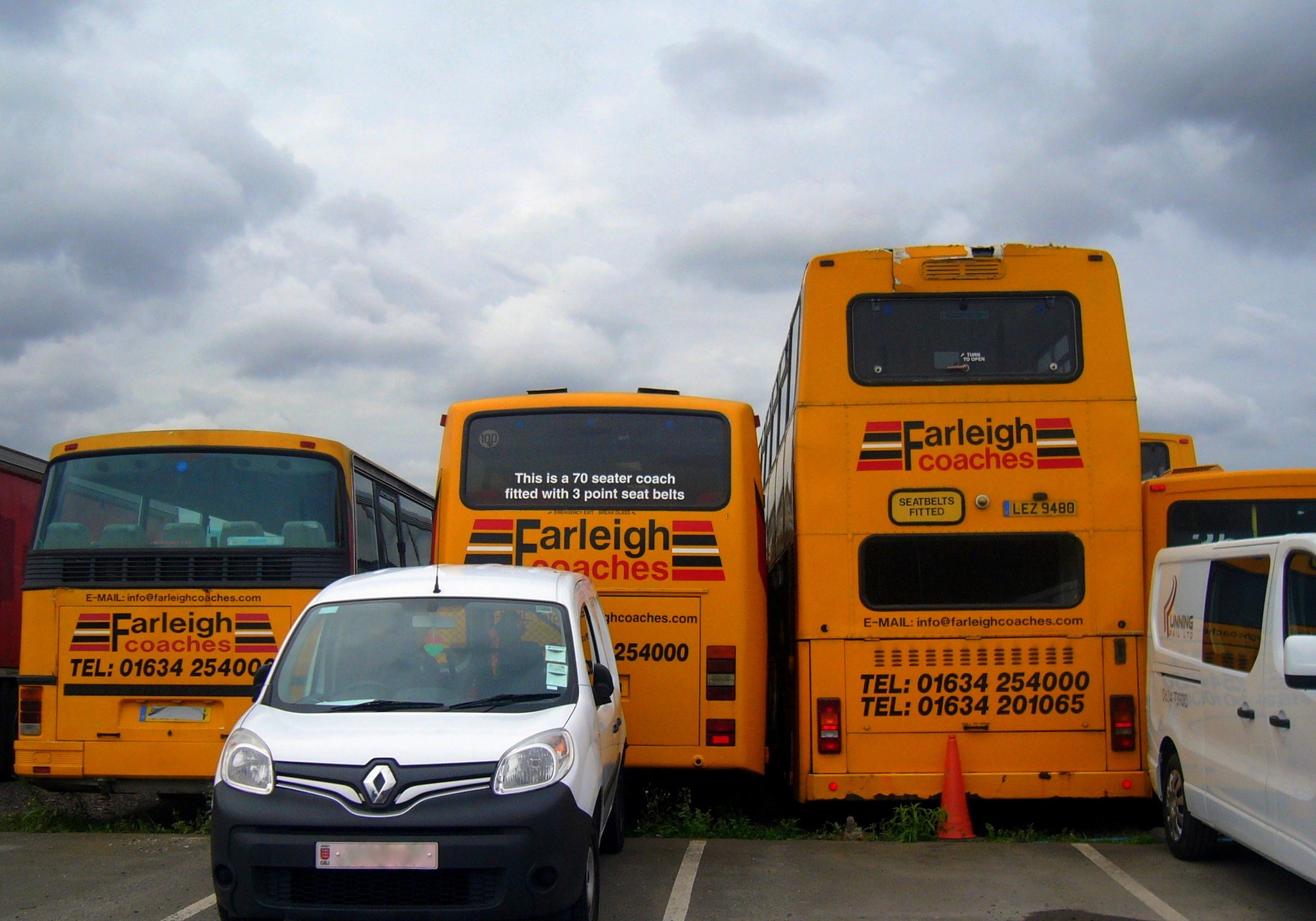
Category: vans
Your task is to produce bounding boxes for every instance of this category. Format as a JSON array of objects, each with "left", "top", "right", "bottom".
[
  {"left": 1147, "top": 533, "right": 1316, "bottom": 894},
  {"left": 212, "top": 564, "right": 630, "bottom": 921}
]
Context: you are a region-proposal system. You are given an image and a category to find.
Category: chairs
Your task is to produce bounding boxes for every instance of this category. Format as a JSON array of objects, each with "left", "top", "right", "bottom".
[
  {"left": 217, "top": 522, "right": 264, "bottom": 546},
  {"left": 43, "top": 521, "right": 94, "bottom": 548},
  {"left": 160, "top": 522, "right": 206, "bottom": 546},
  {"left": 280, "top": 520, "right": 326, "bottom": 549},
  {"left": 101, "top": 523, "right": 147, "bottom": 546},
  {"left": 490, "top": 642, "right": 557, "bottom": 694}
]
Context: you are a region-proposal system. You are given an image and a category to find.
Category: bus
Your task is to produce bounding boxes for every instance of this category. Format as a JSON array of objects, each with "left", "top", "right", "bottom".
[
  {"left": 1137, "top": 430, "right": 1315, "bottom": 605},
  {"left": 431, "top": 387, "right": 771, "bottom": 807},
  {"left": 13, "top": 430, "right": 437, "bottom": 816},
  {"left": 757, "top": 243, "right": 1144, "bottom": 828}
]
